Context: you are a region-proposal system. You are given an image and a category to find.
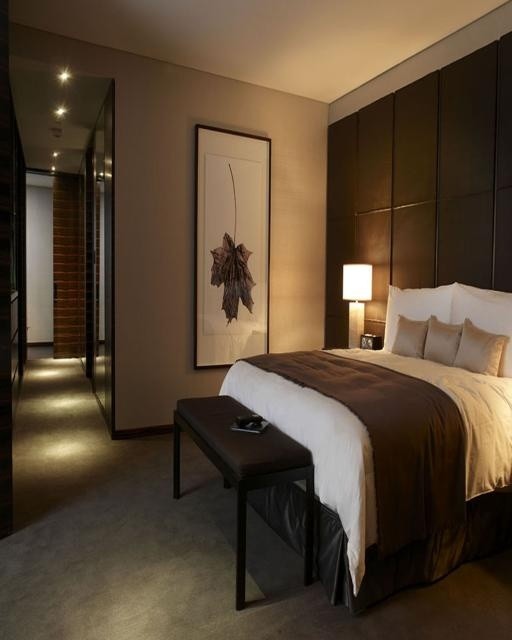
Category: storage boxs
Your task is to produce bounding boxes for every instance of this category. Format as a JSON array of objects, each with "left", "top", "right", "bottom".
[{"left": 173, "top": 395, "right": 314, "bottom": 613}]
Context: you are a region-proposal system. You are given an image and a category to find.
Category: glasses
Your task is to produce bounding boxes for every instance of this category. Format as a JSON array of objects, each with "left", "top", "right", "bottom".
[{"left": 342, "top": 262, "right": 373, "bottom": 351}]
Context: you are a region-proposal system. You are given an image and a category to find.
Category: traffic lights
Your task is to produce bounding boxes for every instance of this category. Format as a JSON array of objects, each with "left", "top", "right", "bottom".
[{"left": 391, "top": 315, "right": 508, "bottom": 377}]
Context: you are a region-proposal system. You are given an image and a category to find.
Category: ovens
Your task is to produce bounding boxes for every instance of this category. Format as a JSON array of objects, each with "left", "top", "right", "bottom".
[{"left": 218, "top": 281, "right": 512, "bottom": 613}]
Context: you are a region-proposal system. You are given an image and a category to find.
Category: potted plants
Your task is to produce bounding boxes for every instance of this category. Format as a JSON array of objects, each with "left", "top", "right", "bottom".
[{"left": 194, "top": 123, "right": 271, "bottom": 370}]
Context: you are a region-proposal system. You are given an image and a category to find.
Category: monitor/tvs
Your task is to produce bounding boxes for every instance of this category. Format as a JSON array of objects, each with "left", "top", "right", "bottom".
[{"left": 229, "top": 414, "right": 270, "bottom": 434}]
